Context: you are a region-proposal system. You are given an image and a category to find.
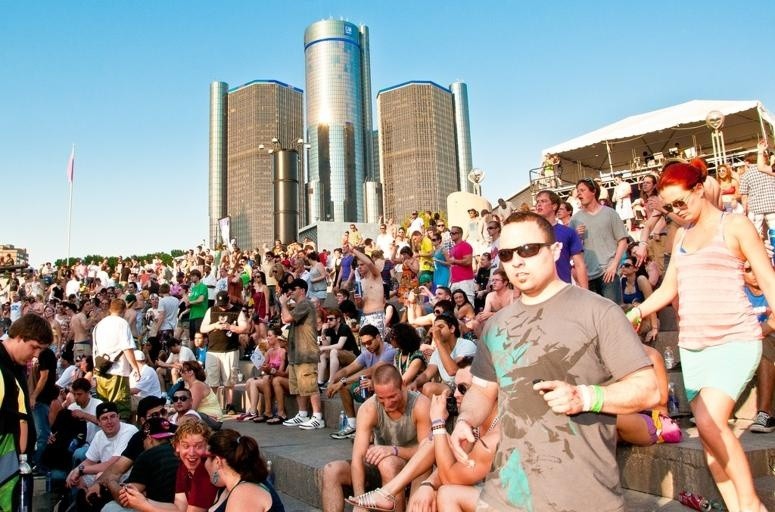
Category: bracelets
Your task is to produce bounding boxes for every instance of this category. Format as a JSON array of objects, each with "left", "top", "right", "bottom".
[
  {"left": 394, "top": 447, "right": 398, "bottom": 455},
  {"left": 352, "top": 248, "right": 355, "bottom": 250},
  {"left": 433, "top": 428, "right": 446, "bottom": 434},
  {"left": 640, "top": 241, "right": 647, "bottom": 245},
  {"left": 432, "top": 423, "right": 445, "bottom": 430},
  {"left": 666, "top": 212, "right": 669, "bottom": 215},
  {"left": 433, "top": 419, "right": 445, "bottom": 424},
  {"left": 422, "top": 482, "right": 436, "bottom": 491},
  {"left": 581, "top": 385, "right": 590, "bottom": 413},
  {"left": 592, "top": 384, "right": 604, "bottom": 413},
  {"left": 635, "top": 307, "right": 642, "bottom": 318},
  {"left": 458, "top": 419, "right": 474, "bottom": 429}
]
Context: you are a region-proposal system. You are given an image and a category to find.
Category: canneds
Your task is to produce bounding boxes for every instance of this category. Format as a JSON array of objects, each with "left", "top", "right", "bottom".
[
  {"left": 46, "top": 472, "right": 52, "bottom": 493},
  {"left": 358, "top": 374, "right": 367, "bottom": 399},
  {"left": 353, "top": 280, "right": 363, "bottom": 297}
]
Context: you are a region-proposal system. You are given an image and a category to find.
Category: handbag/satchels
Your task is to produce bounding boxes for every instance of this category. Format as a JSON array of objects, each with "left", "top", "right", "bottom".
[{"left": 96, "top": 354, "right": 113, "bottom": 374}]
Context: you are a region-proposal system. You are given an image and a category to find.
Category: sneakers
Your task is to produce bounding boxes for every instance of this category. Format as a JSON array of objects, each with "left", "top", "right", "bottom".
[
  {"left": 226, "top": 410, "right": 325, "bottom": 430},
  {"left": 749, "top": 410, "right": 775, "bottom": 433},
  {"left": 329, "top": 425, "right": 356, "bottom": 440}
]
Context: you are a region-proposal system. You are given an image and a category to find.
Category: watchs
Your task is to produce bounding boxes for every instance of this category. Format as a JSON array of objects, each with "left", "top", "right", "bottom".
[
  {"left": 341, "top": 378, "right": 347, "bottom": 386},
  {"left": 79, "top": 466, "right": 84, "bottom": 476}
]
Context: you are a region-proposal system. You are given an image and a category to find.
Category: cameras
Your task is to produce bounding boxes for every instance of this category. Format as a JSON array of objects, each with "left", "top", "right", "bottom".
[{"left": 351, "top": 256, "right": 357, "bottom": 270}]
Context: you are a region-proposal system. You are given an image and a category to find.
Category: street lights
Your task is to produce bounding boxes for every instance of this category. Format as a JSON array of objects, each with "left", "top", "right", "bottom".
[{"left": 257, "top": 137, "right": 311, "bottom": 245}]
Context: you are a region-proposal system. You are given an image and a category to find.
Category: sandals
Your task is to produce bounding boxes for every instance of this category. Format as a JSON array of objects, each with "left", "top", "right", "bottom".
[{"left": 344, "top": 487, "right": 397, "bottom": 512}]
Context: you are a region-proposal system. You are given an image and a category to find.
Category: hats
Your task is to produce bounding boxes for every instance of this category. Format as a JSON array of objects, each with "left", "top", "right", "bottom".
[
  {"left": 216, "top": 291, "right": 229, "bottom": 306},
  {"left": 284, "top": 279, "right": 308, "bottom": 290},
  {"left": 133, "top": 349, "right": 146, "bottom": 361},
  {"left": 96, "top": 395, "right": 176, "bottom": 439}
]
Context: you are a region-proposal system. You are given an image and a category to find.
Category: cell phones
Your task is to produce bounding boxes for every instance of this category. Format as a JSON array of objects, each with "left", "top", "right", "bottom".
[{"left": 532, "top": 379, "right": 552, "bottom": 394}]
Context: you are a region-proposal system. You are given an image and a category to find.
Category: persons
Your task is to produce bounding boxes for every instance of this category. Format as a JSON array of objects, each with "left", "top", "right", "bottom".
[
  {"left": 0, "top": 141, "right": 775, "bottom": 512},
  {"left": 625, "top": 158, "right": 775, "bottom": 512},
  {"left": 449, "top": 212, "right": 661, "bottom": 512}
]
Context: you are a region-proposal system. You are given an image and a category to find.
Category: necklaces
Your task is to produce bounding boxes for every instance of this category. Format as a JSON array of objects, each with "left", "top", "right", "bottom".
[{"left": 475, "top": 417, "right": 499, "bottom": 443}]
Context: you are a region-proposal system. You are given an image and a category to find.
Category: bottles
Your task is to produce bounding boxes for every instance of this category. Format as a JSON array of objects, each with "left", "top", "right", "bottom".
[
  {"left": 264, "top": 461, "right": 275, "bottom": 485},
  {"left": 45, "top": 472, "right": 56, "bottom": 492},
  {"left": 700, "top": 494, "right": 723, "bottom": 511},
  {"left": 18, "top": 454, "right": 33, "bottom": 512},
  {"left": 226, "top": 320, "right": 237, "bottom": 338},
  {"left": 662, "top": 345, "right": 673, "bottom": 370},
  {"left": 339, "top": 411, "right": 347, "bottom": 431}
]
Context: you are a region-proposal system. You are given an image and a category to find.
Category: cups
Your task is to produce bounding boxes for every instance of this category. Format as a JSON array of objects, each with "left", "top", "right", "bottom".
[
  {"left": 238, "top": 373, "right": 244, "bottom": 383},
  {"left": 354, "top": 296, "right": 362, "bottom": 308}
]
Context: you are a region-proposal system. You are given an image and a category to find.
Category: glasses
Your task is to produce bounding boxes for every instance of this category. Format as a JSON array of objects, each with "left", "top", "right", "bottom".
[
  {"left": 438, "top": 225, "right": 444, "bottom": 227},
  {"left": 457, "top": 384, "right": 470, "bottom": 395},
  {"left": 450, "top": 232, "right": 457, "bottom": 235},
  {"left": 662, "top": 186, "right": 695, "bottom": 213},
  {"left": 488, "top": 226, "right": 497, "bottom": 230},
  {"left": 622, "top": 262, "right": 634, "bottom": 268},
  {"left": 496, "top": 241, "right": 553, "bottom": 263},
  {"left": 327, "top": 318, "right": 336, "bottom": 321},
  {"left": 362, "top": 337, "right": 376, "bottom": 346},
  {"left": 432, "top": 239, "right": 436, "bottom": 241},
  {"left": 172, "top": 395, "right": 190, "bottom": 402}
]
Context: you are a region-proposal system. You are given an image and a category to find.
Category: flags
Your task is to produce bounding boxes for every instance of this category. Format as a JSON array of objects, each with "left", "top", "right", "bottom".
[{"left": 68, "top": 151, "right": 75, "bottom": 182}]
